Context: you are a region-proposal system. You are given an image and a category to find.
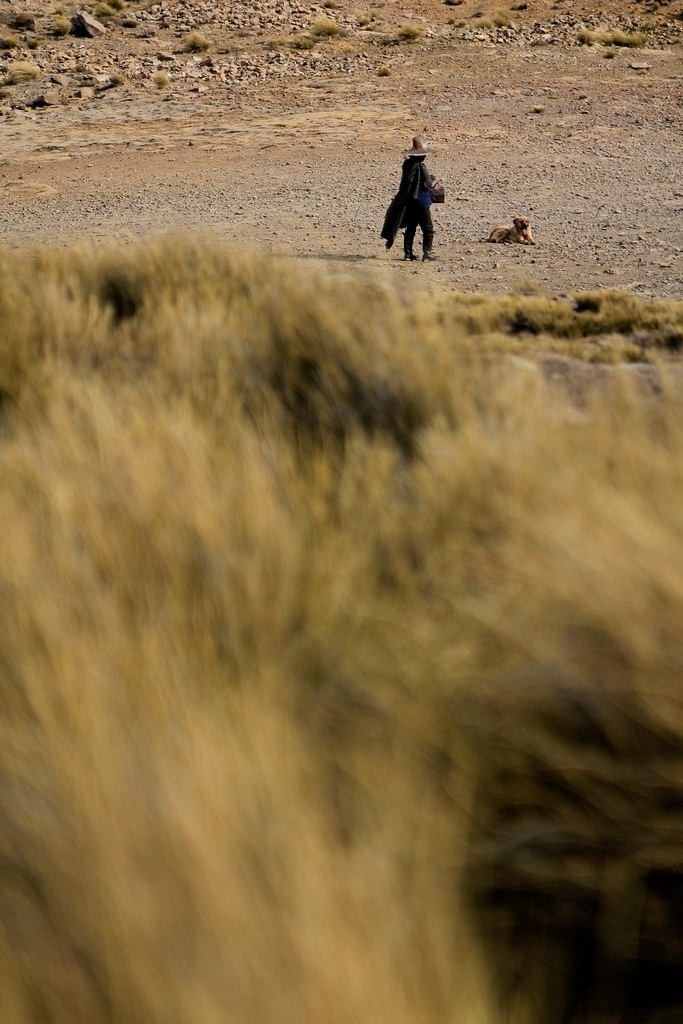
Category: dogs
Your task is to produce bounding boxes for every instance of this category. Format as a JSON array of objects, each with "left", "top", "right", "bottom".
[{"left": 489, "top": 215, "right": 536, "bottom": 245}]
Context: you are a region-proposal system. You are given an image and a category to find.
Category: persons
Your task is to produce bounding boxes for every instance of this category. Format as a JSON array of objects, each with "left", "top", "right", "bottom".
[{"left": 380, "top": 137, "right": 444, "bottom": 262}]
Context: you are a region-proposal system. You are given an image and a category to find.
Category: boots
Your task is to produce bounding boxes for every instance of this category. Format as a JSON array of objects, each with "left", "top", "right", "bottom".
[
  {"left": 422, "top": 237, "right": 440, "bottom": 261},
  {"left": 404, "top": 234, "right": 420, "bottom": 261}
]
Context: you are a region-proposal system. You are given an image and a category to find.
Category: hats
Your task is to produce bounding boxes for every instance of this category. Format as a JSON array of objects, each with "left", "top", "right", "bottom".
[{"left": 405, "top": 136, "right": 431, "bottom": 156}]
